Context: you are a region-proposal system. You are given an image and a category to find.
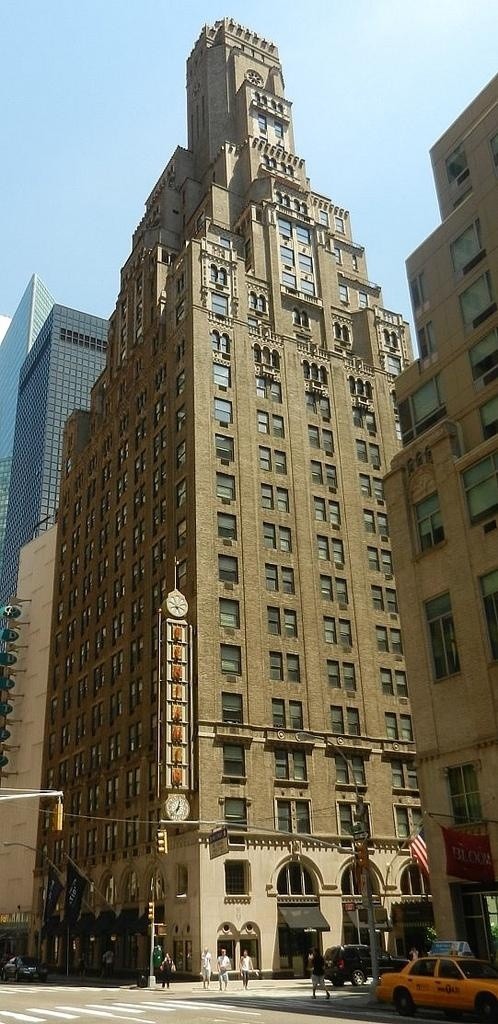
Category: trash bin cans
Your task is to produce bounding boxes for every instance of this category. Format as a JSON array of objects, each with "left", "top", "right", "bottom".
[{"left": 137, "top": 967, "right": 148, "bottom": 988}]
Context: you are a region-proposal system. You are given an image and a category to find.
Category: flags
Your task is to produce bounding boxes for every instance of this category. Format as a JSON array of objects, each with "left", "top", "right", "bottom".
[
  {"left": 410, "top": 825, "right": 429, "bottom": 873},
  {"left": 64, "top": 857, "right": 87, "bottom": 928},
  {"left": 45, "top": 865, "right": 63, "bottom": 921}
]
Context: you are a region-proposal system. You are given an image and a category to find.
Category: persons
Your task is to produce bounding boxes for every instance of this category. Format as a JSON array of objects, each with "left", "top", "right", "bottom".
[
  {"left": 201, "top": 947, "right": 212, "bottom": 989},
  {"left": 161, "top": 957, "right": 171, "bottom": 989},
  {"left": 240, "top": 949, "right": 253, "bottom": 990},
  {"left": 79, "top": 951, "right": 87, "bottom": 979},
  {"left": 409, "top": 946, "right": 419, "bottom": 961},
  {"left": 218, "top": 949, "right": 229, "bottom": 991},
  {"left": 102, "top": 947, "right": 114, "bottom": 976},
  {"left": 311, "top": 948, "right": 330, "bottom": 999}
]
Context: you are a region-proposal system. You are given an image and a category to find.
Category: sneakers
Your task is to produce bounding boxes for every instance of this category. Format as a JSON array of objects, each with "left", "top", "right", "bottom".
[{"left": 312, "top": 994, "right": 330, "bottom": 999}]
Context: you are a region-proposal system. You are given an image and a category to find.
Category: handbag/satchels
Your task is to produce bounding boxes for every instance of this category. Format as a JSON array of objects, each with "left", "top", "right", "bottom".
[
  {"left": 221, "top": 964, "right": 232, "bottom": 970},
  {"left": 171, "top": 965, "right": 176, "bottom": 973}
]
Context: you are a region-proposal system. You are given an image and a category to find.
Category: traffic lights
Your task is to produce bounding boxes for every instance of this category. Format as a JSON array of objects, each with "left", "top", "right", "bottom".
[
  {"left": 156, "top": 829, "right": 167, "bottom": 854},
  {"left": 356, "top": 839, "right": 366, "bottom": 869},
  {"left": 148, "top": 902, "right": 155, "bottom": 920},
  {"left": 156, "top": 925, "right": 167, "bottom": 935}
]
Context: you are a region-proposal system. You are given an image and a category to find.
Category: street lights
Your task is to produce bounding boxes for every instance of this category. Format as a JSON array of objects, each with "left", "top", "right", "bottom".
[
  {"left": 295, "top": 732, "right": 379, "bottom": 981},
  {"left": 3, "top": 842, "right": 46, "bottom": 962}
]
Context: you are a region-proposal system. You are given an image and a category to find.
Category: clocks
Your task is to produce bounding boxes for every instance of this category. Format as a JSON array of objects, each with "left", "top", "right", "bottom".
[{"left": 166, "top": 793, "right": 191, "bottom": 821}]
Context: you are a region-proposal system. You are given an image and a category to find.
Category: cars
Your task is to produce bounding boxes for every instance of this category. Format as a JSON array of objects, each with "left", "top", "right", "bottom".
[
  {"left": 324, "top": 941, "right": 498, "bottom": 1019},
  {"left": 2, "top": 956, "right": 48, "bottom": 981}
]
